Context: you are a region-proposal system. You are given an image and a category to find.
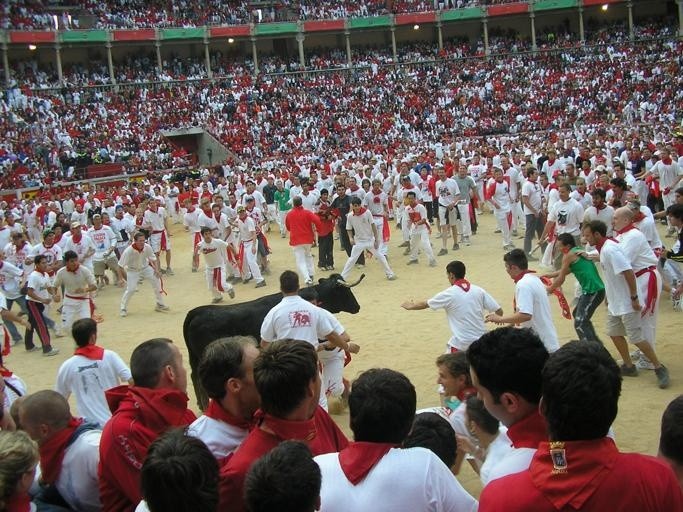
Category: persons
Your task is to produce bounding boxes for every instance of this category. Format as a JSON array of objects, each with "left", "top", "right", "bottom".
[{"left": 1, "top": 0, "right": 683, "bottom": 512}]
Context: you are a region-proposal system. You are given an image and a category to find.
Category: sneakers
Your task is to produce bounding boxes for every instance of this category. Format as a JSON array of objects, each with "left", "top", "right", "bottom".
[
  {"left": 120, "top": 308, "right": 127, "bottom": 316},
  {"left": 227, "top": 274, "right": 266, "bottom": 287},
  {"left": 401, "top": 241, "right": 417, "bottom": 265},
  {"left": 526, "top": 252, "right": 538, "bottom": 262},
  {"left": 155, "top": 303, "right": 168, "bottom": 310},
  {"left": 228, "top": 289, "right": 235, "bottom": 299},
  {"left": 618, "top": 351, "right": 668, "bottom": 388},
  {"left": 672, "top": 290, "right": 680, "bottom": 310},
  {"left": 28, "top": 345, "right": 41, "bottom": 351},
  {"left": 434, "top": 232, "right": 471, "bottom": 255},
  {"left": 503, "top": 244, "right": 515, "bottom": 250},
  {"left": 43, "top": 349, "right": 59, "bottom": 356},
  {"left": 212, "top": 297, "right": 223, "bottom": 303}
]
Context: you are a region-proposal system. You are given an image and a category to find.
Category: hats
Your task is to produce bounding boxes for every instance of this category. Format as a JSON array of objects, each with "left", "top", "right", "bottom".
[
  {"left": 403, "top": 175, "right": 410, "bottom": 180},
  {"left": 237, "top": 206, "right": 245, "bottom": 213},
  {"left": 595, "top": 165, "right": 605, "bottom": 171},
  {"left": 42, "top": 229, "right": 53, "bottom": 238},
  {"left": 70, "top": 221, "right": 82, "bottom": 229}
]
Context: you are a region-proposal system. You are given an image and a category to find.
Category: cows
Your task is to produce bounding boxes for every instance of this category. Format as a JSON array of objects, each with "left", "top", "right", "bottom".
[{"left": 182, "top": 272, "right": 367, "bottom": 411}]
